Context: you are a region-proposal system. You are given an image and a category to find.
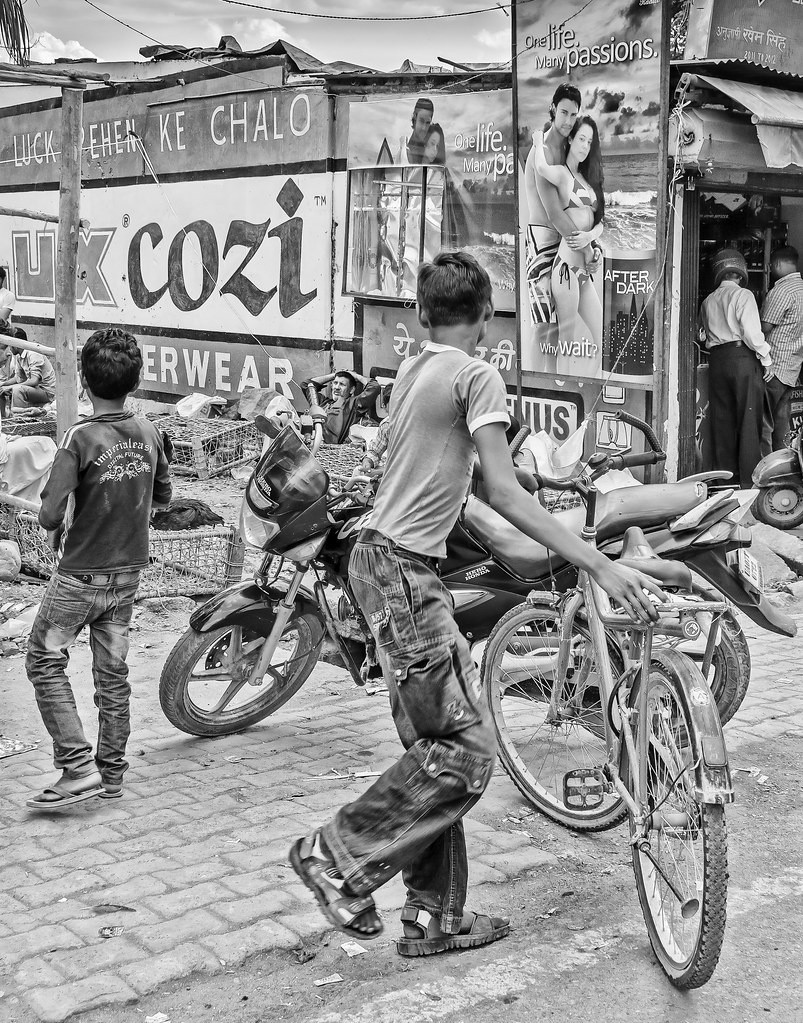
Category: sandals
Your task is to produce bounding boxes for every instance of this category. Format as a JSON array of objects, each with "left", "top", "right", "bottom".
[
  {"left": 397, "top": 906, "right": 510, "bottom": 956},
  {"left": 289, "top": 827, "right": 383, "bottom": 940}
]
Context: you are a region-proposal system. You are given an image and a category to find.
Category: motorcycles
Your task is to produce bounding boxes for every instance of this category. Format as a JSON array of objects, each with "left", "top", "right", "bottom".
[
  {"left": 749, "top": 412, "right": 803, "bottom": 528},
  {"left": 160, "top": 411, "right": 799, "bottom": 738}
]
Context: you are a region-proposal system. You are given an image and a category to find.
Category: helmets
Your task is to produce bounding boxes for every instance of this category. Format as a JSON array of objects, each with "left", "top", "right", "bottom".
[{"left": 710, "top": 249, "right": 749, "bottom": 288}]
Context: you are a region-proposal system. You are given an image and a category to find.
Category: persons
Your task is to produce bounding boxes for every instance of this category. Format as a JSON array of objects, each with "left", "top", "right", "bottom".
[
  {"left": 699, "top": 247, "right": 803, "bottom": 492},
  {"left": 287, "top": 251, "right": 672, "bottom": 953},
  {"left": 23, "top": 327, "right": 172, "bottom": 807},
  {"left": 0, "top": 266, "right": 55, "bottom": 415},
  {"left": 253, "top": 368, "right": 384, "bottom": 446},
  {"left": 356, "top": 381, "right": 395, "bottom": 473},
  {"left": 525, "top": 82, "right": 606, "bottom": 381},
  {"left": 395, "top": 98, "right": 446, "bottom": 262}
]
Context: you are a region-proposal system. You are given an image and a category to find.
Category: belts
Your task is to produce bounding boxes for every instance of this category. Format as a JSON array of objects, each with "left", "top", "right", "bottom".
[
  {"left": 714, "top": 340, "right": 746, "bottom": 348},
  {"left": 73, "top": 569, "right": 141, "bottom": 586}
]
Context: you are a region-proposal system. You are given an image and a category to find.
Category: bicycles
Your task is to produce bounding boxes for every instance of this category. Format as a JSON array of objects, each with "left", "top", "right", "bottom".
[{"left": 479, "top": 414, "right": 735, "bottom": 987}]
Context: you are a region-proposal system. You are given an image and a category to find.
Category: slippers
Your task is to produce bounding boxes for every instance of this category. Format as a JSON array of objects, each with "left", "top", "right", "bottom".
[
  {"left": 97, "top": 785, "right": 123, "bottom": 797},
  {"left": 26, "top": 787, "right": 106, "bottom": 808}
]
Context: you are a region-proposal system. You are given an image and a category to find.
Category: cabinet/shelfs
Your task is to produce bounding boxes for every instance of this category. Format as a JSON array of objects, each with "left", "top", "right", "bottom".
[{"left": 699, "top": 214, "right": 791, "bottom": 325}]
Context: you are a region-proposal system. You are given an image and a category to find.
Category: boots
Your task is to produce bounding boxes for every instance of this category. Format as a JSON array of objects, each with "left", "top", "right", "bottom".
[{"left": 255, "top": 415, "right": 280, "bottom": 439}]
